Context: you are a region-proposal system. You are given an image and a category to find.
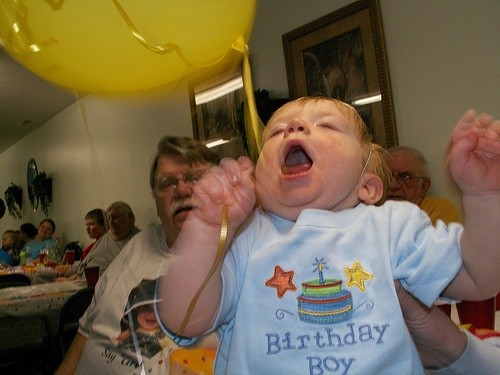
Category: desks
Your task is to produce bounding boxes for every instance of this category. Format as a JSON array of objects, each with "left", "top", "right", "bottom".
[{"left": 0, "top": 279, "right": 88, "bottom": 348}]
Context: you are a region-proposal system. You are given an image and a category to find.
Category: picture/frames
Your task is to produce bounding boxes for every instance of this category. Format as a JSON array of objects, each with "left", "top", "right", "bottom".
[
  {"left": 281, "top": 0, "right": 398, "bottom": 151},
  {"left": 188, "top": 57, "right": 258, "bottom": 164}
]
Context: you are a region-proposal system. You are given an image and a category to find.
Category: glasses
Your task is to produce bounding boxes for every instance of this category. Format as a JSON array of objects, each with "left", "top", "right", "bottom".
[
  {"left": 387, "top": 174, "right": 424, "bottom": 183},
  {"left": 151, "top": 174, "right": 203, "bottom": 193}
]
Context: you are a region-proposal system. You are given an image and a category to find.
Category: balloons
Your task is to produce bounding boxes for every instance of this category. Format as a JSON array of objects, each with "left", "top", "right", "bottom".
[{"left": 0, "top": 0, "right": 255, "bottom": 92}]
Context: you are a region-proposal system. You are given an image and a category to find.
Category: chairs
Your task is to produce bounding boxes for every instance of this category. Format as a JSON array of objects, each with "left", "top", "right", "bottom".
[{"left": 58, "top": 287, "right": 95, "bottom": 358}]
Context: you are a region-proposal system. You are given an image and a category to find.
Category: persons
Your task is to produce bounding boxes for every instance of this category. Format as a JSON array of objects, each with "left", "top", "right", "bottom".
[
  {"left": 80, "top": 201, "right": 143, "bottom": 277},
  {"left": 394, "top": 280, "right": 500, "bottom": 375},
  {"left": 53, "top": 136, "right": 218, "bottom": 375},
  {"left": 382, "top": 147, "right": 466, "bottom": 228},
  {"left": 155, "top": 97, "right": 500, "bottom": 375},
  {"left": 0, "top": 219, "right": 55, "bottom": 267}
]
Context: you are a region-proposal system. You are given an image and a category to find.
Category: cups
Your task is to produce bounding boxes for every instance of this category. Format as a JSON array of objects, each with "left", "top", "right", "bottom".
[
  {"left": 39, "top": 254, "right": 46, "bottom": 263},
  {"left": 66, "top": 250, "right": 75, "bottom": 265},
  {"left": 84, "top": 266, "right": 100, "bottom": 288},
  {"left": 19, "top": 252, "right": 26, "bottom": 264}
]
